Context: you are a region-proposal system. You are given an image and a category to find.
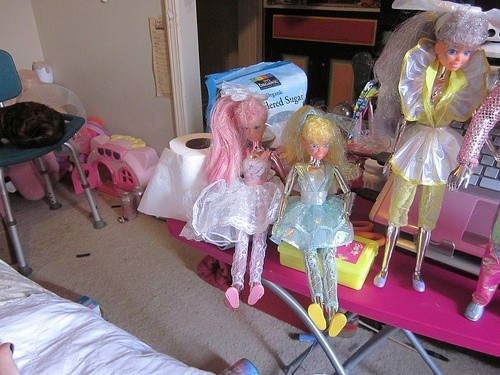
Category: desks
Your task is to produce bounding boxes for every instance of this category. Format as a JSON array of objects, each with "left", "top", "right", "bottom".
[{"left": 167, "top": 217, "right": 500, "bottom": 375}]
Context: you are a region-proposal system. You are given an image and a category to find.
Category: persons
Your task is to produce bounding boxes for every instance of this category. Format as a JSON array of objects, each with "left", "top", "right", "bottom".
[
  {"left": 447, "top": 84, "right": 500, "bottom": 322},
  {"left": 191, "top": 87, "right": 288, "bottom": 310},
  {"left": 372, "top": 0, "right": 500, "bottom": 292},
  {"left": 269, "top": 105, "right": 364, "bottom": 337}
]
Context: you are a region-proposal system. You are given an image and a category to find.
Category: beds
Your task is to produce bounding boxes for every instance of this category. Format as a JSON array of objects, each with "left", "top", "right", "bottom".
[{"left": 0, "top": 259, "right": 214, "bottom": 375}]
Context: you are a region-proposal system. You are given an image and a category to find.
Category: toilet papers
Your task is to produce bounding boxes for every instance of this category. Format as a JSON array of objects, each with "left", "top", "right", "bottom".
[{"left": 137, "top": 132, "right": 214, "bottom": 221}]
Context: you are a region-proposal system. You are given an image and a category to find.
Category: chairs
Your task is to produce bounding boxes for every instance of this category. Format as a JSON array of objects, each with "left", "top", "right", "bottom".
[{"left": 0, "top": 48, "right": 107, "bottom": 278}]
